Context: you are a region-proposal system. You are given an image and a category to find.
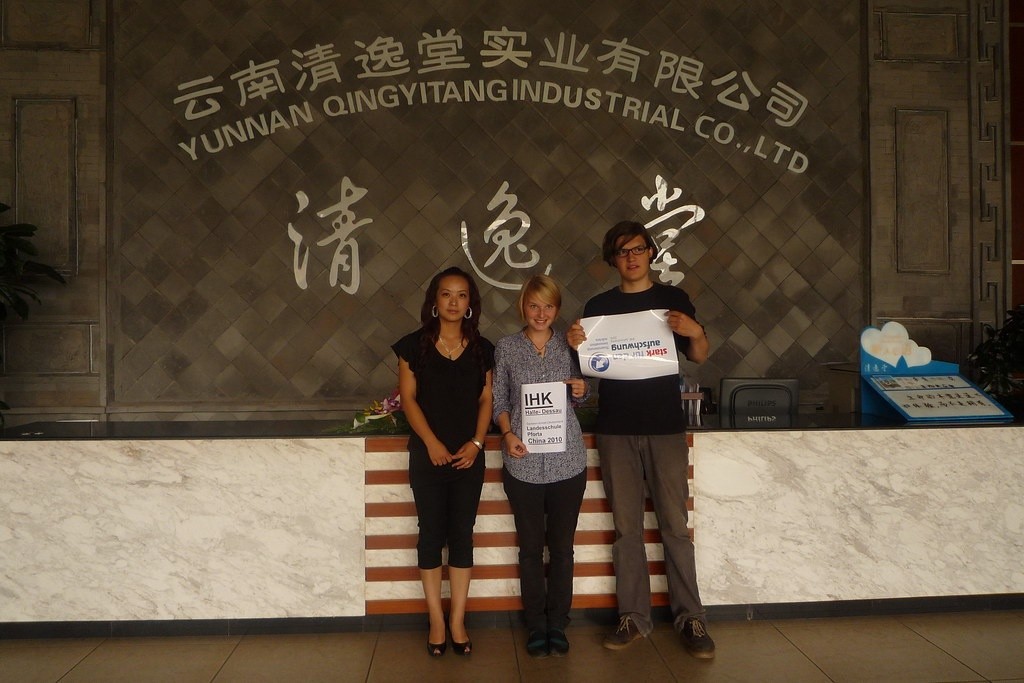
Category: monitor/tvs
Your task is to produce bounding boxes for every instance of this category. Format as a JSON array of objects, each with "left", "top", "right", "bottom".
[{"left": 720, "top": 379, "right": 799, "bottom": 430}]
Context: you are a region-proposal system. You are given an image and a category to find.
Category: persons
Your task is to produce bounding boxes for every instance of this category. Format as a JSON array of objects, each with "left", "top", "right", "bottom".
[
  {"left": 491, "top": 276, "right": 591, "bottom": 660},
  {"left": 391, "top": 266, "right": 496, "bottom": 656},
  {"left": 568, "top": 221, "right": 716, "bottom": 660}
]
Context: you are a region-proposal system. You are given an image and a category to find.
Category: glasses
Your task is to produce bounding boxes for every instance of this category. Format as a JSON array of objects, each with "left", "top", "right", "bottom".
[{"left": 614, "top": 245, "right": 649, "bottom": 258}]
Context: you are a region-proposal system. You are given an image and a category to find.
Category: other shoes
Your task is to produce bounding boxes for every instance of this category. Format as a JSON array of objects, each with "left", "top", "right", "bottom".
[
  {"left": 602, "top": 615, "right": 644, "bottom": 650},
  {"left": 427, "top": 621, "right": 446, "bottom": 658},
  {"left": 448, "top": 619, "right": 472, "bottom": 656},
  {"left": 549, "top": 627, "right": 570, "bottom": 658},
  {"left": 679, "top": 617, "right": 716, "bottom": 659},
  {"left": 526, "top": 629, "right": 550, "bottom": 660}
]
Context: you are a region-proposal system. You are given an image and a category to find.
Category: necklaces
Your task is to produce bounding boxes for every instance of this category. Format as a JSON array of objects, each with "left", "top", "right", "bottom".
[
  {"left": 524, "top": 330, "right": 552, "bottom": 356},
  {"left": 438, "top": 335, "right": 462, "bottom": 359}
]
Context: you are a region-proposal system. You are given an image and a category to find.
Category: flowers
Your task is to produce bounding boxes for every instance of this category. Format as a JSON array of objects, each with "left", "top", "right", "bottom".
[{"left": 320, "top": 391, "right": 408, "bottom": 435}]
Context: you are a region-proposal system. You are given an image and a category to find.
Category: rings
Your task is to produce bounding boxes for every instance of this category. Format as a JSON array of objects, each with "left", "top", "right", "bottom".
[{"left": 465, "top": 461, "right": 471, "bottom": 465}]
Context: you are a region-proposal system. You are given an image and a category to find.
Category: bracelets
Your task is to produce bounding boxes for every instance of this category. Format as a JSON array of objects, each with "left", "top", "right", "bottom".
[
  {"left": 471, "top": 438, "right": 482, "bottom": 450},
  {"left": 503, "top": 431, "right": 512, "bottom": 440}
]
